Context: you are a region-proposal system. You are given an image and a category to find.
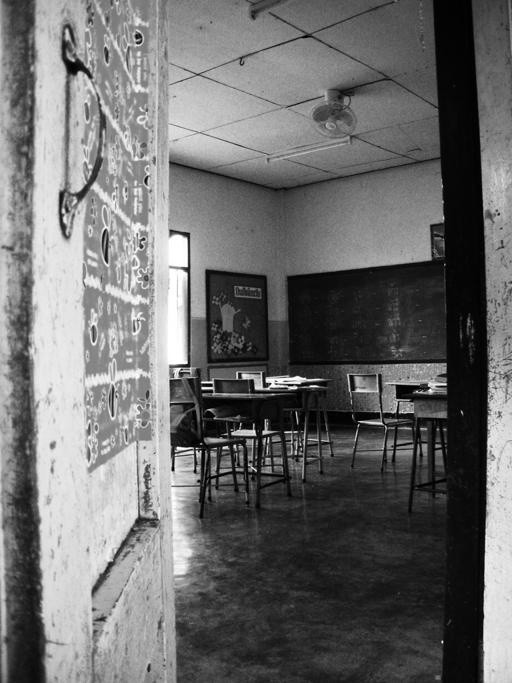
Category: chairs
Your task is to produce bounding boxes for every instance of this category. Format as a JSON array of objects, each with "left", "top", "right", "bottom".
[
  {"left": 347, "top": 372, "right": 424, "bottom": 473},
  {"left": 168, "top": 366, "right": 301, "bottom": 518}
]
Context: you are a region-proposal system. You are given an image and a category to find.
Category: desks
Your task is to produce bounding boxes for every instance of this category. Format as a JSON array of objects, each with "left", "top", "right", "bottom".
[
  {"left": 386, "top": 376, "right": 448, "bottom": 516},
  {"left": 268, "top": 375, "right": 336, "bottom": 483}
]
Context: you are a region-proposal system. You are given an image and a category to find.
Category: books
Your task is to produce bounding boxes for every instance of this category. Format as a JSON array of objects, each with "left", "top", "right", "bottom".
[
  {"left": 428, "top": 374, "right": 447, "bottom": 394},
  {"left": 204, "top": 405, "right": 251, "bottom": 423}
]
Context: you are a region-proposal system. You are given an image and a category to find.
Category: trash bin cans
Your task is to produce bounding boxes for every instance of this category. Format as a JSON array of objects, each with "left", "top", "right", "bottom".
[{"left": 174, "top": 368, "right": 201, "bottom": 436}]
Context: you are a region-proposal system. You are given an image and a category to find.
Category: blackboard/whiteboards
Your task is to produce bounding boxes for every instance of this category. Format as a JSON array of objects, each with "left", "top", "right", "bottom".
[{"left": 287, "top": 260, "right": 445, "bottom": 364}]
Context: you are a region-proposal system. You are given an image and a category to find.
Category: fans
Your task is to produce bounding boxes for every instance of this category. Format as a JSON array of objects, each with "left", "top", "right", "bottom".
[{"left": 308, "top": 89, "right": 357, "bottom": 140}]
